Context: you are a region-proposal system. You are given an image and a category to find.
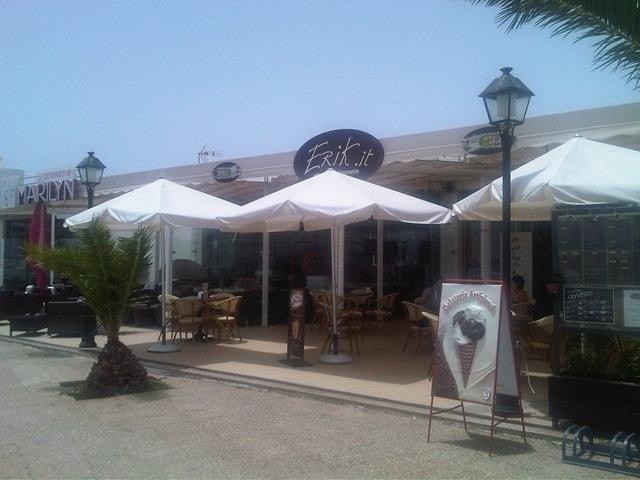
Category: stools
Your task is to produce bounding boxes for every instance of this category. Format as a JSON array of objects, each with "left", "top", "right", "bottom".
[{"left": 8, "top": 313, "right": 47, "bottom": 336}]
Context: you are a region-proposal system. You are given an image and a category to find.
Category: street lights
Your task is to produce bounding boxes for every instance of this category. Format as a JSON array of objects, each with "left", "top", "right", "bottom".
[
  {"left": 76, "top": 151, "right": 106, "bottom": 346},
  {"left": 480, "top": 67, "right": 535, "bottom": 419}
]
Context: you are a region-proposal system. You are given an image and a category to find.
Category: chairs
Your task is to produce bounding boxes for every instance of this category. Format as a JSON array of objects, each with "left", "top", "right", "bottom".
[
  {"left": 308, "top": 286, "right": 554, "bottom": 394},
  {"left": 157, "top": 288, "right": 243, "bottom": 344}
]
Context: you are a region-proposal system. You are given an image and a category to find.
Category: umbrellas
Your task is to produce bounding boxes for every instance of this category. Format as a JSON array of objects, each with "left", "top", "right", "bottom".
[
  {"left": 62, "top": 178, "right": 239, "bottom": 353},
  {"left": 212, "top": 166, "right": 451, "bottom": 365},
  {"left": 453, "top": 134, "right": 640, "bottom": 355}
]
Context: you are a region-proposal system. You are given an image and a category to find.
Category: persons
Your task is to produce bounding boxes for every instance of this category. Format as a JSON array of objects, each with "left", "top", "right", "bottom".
[
  {"left": 511, "top": 274, "right": 529, "bottom": 305},
  {"left": 415, "top": 274, "right": 444, "bottom": 327}
]
